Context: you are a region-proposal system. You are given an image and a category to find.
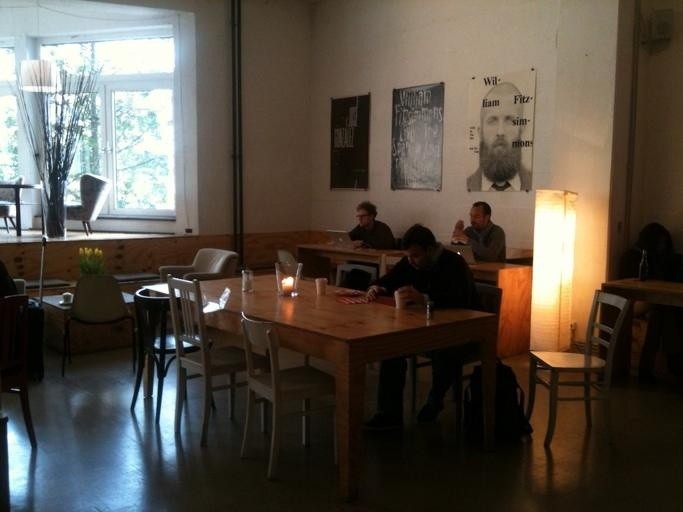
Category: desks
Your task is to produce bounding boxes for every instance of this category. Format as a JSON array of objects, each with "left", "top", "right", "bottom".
[
  {"left": 601, "top": 277, "right": 682, "bottom": 387},
  {"left": 0, "top": 183, "right": 34, "bottom": 236},
  {"left": 296, "top": 243, "right": 532, "bottom": 356},
  {"left": 31, "top": 291, "right": 137, "bottom": 377},
  {"left": 145, "top": 269, "right": 500, "bottom": 500}
]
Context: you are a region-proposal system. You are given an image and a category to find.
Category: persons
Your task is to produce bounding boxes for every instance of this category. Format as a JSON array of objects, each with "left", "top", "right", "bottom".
[
  {"left": 349, "top": 201, "right": 394, "bottom": 250},
  {"left": 618, "top": 222, "right": 683, "bottom": 385},
  {"left": 360, "top": 224, "right": 481, "bottom": 431},
  {"left": 451, "top": 202, "right": 506, "bottom": 263},
  {"left": 466, "top": 82, "right": 532, "bottom": 191}
]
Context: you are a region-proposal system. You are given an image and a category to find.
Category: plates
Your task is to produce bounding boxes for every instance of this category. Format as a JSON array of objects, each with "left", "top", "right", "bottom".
[{"left": 58, "top": 300, "right": 72, "bottom": 305}]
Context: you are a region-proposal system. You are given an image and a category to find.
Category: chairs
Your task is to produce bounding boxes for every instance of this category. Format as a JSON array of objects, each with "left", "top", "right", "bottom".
[
  {"left": 157, "top": 249, "right": 241, "bottom": 322},
  {"left": 165, "top": 271, "right": 270, "bottom": 439},
  {"left": 239, "top": 309, "right": 335, "bottom": 483},
  {"left": 0, "top": 178, "right": 23, "bottom": 234},
  {"left": 277, "top": 249, "right": 298, "bottom": 273},
  {"left": 67, "top": 174, "right": 110, "bottom": 236},
  {"left": 531, "top": 290, "right": 629, "bottom": 448},
  {"left": 131, "top": 286, "right": 218, "bottom": 423},
  {"left": 303, "top": 263, "right": 379, "bottom": 366},
  {"left": 62, "top": 274, "right": 136, "bottom": 376},
  {"left": 412, "top": 284, "right": 503, "bottom": 452},
  {"left": 1, "top": 295, "right": 35, "bottom": 448}
]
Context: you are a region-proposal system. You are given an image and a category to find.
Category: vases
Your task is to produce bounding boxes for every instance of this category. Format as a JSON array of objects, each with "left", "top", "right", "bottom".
[{"left": 39, "top": 175, "right": 66, "bottom": 237}]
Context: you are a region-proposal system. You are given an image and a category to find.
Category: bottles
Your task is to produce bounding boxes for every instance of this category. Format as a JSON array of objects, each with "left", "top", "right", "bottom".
[
  {"left": 241, "top": 270, "right": 254, "bottom": 294},
  {"left": 425, "top": 300, "right": 433, "bottom": 320},
  {"left": 639, "top": 250, "right": 648, "bottom": 282}
]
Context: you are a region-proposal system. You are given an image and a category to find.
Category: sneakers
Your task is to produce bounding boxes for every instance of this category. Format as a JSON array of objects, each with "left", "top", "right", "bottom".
[
  {"left": 417, "top": 401, "right": 444, "bottom": 421},
  {"left": 364, "top": 414, "right": 403, "bottom": 430}
]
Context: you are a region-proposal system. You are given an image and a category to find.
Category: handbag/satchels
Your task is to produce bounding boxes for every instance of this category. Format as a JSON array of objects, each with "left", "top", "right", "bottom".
[{"left": 465, "top": 363, "right": 532, "bottom": 434}]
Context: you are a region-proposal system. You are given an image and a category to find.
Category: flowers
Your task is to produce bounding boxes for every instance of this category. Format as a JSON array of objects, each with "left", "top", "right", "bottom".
[{"left": 78, "top": 246, "right": 105, "bottom": 276}]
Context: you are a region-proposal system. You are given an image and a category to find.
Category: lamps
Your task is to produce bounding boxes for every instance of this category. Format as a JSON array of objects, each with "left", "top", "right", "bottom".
[
  {"left": 18, "top": 0, "right": 62, "bottom": 92},
  {"left": 530, "top": 189, "right": 578, "bottom": 352}
]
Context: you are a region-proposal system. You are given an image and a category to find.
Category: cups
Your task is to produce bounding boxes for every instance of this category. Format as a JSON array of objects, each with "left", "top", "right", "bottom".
[
  {"left": 62, "top": 292, "right": 72, "bottom": 303},
  {"left": 394, "top": 290, "right": 406, "bottom": 309},
  {"left": 314, "top": 278, "right": 327, "bottom": 295}
]
